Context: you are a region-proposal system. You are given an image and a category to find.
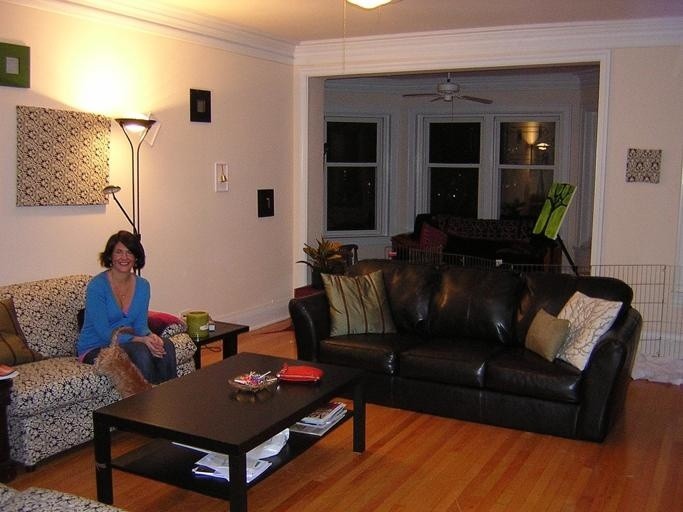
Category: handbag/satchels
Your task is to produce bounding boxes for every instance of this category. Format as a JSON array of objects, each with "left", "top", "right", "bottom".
[{"left": 277, "top": 364, "right": 323, "bottom": 383}]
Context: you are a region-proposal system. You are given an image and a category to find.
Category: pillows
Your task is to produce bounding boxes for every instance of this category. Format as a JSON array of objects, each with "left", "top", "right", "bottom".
[
  {"left": 557, "top": 291, "right": 624, "bottom": 375},
  {"left": 319, "top": 269, "right": 397, "bottom": 338},
  {"left": 417, "top": 222, "right": 447, "bottom": 253},
  {"left": 521, "top": 307, "right": 572, "bottom": 363},
  {"left": 0, "top": 296, "right": 48, "bottom": 366}
]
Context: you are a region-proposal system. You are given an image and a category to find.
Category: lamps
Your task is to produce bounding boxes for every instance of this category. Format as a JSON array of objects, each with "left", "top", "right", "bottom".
[
  {"left": 101, "top": 118, "right": 158, "bottom": 278},
  {"left": 521, "top": 122, "right": 539, "bottom": 145}
]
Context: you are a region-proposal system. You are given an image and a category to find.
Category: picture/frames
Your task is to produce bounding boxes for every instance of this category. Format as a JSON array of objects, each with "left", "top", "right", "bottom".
[
  {"left": 214, "top": 161, "right": 228, "bottom": 192},
  {"left": 188, "top": 88, "right": 212, "bottom": 125},
  {"left": 0, "top": 42, "right": 30, "bottom": 88},
  {"left": 256, "top": 188, "right": 274, "bottom": 219}
]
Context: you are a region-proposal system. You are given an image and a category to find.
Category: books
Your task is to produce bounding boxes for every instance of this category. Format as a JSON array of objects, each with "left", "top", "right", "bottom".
[{"left": 171, "top": 402, "right": 347, "bottom": 485}]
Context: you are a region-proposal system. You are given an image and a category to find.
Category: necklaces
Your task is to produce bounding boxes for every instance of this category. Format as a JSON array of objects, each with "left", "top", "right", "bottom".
[{"left": 111, "top": 274, "right": 130, "bottom": 300}]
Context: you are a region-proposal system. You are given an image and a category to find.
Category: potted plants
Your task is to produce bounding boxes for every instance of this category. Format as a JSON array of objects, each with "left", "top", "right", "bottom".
[{"left": 296, "top": 238, "right": 348, "bottom": 289}]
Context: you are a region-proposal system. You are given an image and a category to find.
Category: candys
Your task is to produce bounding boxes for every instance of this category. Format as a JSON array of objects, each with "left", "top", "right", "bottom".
[{"left": 233, "top": 370, "right": 277, "bottom": 387}]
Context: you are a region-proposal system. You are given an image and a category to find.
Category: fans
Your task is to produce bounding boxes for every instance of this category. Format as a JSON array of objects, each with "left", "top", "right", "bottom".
[{"left": 402, "top": 72, "right": 494, "bottom": 108}]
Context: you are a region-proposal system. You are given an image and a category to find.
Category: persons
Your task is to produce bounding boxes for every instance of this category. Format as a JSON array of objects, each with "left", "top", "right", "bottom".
[{"left": 75, "top": 231, "right": 176, "bottom": 385}]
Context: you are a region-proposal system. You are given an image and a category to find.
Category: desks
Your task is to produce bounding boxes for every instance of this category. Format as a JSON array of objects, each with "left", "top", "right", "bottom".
[{"left": 185, "top": 317, "right": 248, "bottom": 368}]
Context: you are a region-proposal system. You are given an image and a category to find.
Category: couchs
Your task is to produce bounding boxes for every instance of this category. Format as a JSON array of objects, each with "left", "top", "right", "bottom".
[
  {"left": 390, "top": 214, "right": 562, "bottom": 274},
  {"left": 0, "top": 482, "right": 124, "bottom": 510},
  {"left": 0, "top": 274, "right": 197, "bottom": 470},
  {"left": 287, "top": 257, "right": 642, "bottom": 444}
]
